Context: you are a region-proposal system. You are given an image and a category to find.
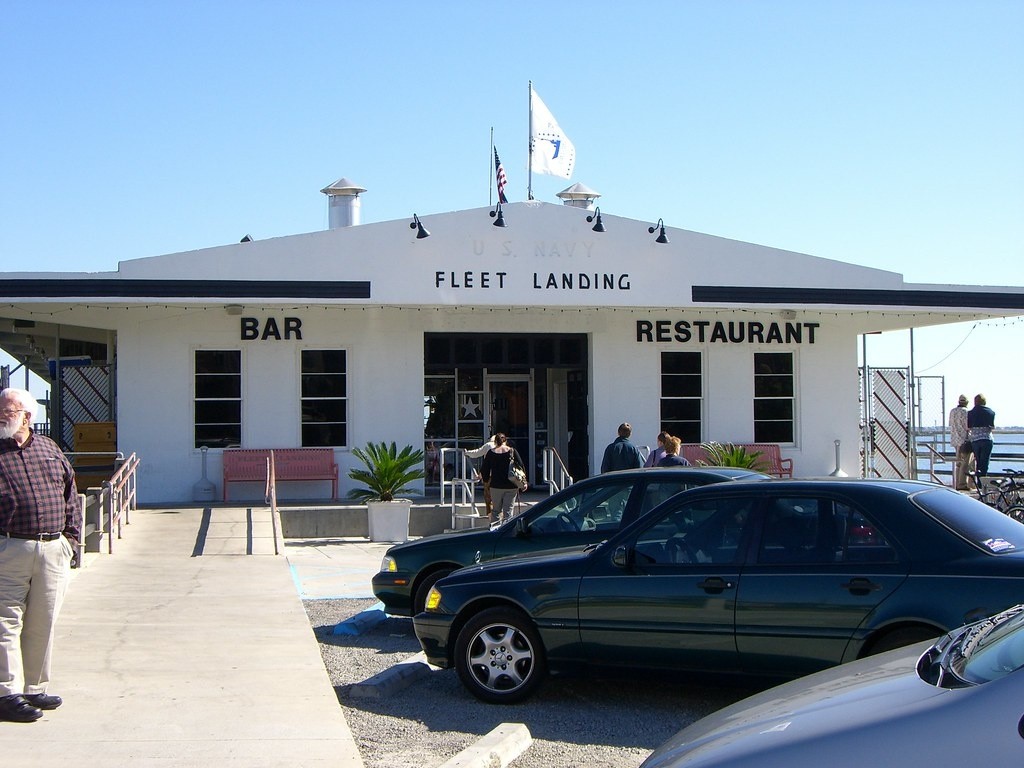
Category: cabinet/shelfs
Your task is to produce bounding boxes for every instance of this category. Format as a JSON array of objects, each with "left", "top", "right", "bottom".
[{"left": 73, "top": 421, "right": 116, "bottom": 452}]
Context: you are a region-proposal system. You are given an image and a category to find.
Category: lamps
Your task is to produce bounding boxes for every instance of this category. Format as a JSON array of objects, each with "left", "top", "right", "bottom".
[
  {"left": 586, "top": 206, "right": 606, "bottom": 232},
  {"left": 12, "top": 320, "right": 48, "bottom": 362},
  {"left": 410, "top": 213, "right": 431, "bottom": 239},
  {"left": 648, "top": 218, "right": 670, "bottom": 243},
  {"left": 490, "top": 202, "right": 508, "bottom": 227}
]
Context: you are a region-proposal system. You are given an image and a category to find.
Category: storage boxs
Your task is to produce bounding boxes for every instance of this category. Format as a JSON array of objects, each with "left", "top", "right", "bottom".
[{"left": 48, "top": 355, "right": 92, "bottom": 381}]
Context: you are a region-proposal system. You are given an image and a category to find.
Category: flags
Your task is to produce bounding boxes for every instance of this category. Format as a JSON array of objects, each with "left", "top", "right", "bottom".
[
  {"left": 495, "top": 147, "right": 508, "bottom": 203},
  {"left": 531, "top": 90, "right": 575, "bottom": 178}
]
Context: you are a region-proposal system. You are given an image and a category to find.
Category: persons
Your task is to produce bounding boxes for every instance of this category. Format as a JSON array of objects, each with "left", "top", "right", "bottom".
[
  {"left": 465, "top": 435, "right": 503, "bottom": 530},
  {"left": 482, "top": 433, "right": 527, "bottom": 527},
  {"left": 949, "top": 394, "right": 970, "bottom": 490},
  {"left": 968, "top": 394, "right": 995, "bottom": 490},
  {"left": 657, "top": 436, "right": 691, "bottom": 505},
  {"left": 644, "top": 432, "right": 670, "bottom": 467},
  {"left": 601, "top": 423, "right": 642, "bottom": 520},
  {"left": 0, "top": 388, "right": 82, "bottom": 722}
]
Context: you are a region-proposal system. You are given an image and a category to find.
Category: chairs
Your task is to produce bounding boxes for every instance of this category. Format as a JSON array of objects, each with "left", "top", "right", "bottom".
[{"left": 732, "top": 505, "right": 804, "bottom": 566}]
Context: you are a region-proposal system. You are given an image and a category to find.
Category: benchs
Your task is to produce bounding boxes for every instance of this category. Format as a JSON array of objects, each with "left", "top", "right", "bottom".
[
  {"left": 223, "top": 448, "right": 339, "bottom": 505},
  {"left": 681, "top": 444, "right": 794, "bottom": 478}
]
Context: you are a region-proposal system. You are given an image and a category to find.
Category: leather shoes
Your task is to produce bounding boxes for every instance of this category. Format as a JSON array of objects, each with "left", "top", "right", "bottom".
[
  {"left": 0, "top": 695, "right": 43, "bottom": 722},
  {"left": 23, "top": 694, "right": 62, "bottom": 709}
]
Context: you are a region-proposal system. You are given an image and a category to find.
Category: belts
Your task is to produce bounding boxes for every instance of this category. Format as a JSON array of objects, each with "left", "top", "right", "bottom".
[{"left": 0, "top": 531, "right": 60, "bottom": 540}]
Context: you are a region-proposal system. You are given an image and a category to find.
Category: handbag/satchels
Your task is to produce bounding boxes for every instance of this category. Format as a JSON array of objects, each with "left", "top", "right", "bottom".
[
  {"left": 508, "top": 448, "right": 526, "bottom": 489},
  {"left": 959, "top": 430, "right": 973, "bottom": 453}
]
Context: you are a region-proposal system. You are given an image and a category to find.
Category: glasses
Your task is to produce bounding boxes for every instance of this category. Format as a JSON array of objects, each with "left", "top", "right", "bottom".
[{"left": 0, "top": 408, "right": 26, "bottom": 416}]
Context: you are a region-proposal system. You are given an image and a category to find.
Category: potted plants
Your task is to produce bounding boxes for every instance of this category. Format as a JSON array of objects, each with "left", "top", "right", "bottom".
[{"left": 346, "top": 439, "right": 431, "bottom": 543}]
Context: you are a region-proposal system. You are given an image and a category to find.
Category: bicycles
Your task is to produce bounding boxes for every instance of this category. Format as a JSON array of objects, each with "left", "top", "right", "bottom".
[{"left": 965, "top": 467, "right": 1024, "bottom": 524}]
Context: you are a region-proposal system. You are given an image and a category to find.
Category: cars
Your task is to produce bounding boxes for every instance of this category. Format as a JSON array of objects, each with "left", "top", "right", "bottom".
[
  {"left": 412, "top": 472, "right": 1024, "bottom": 705},
  {"left": 372, "top": 464, "right": 898, "bottom": 638},
  {"left": 638, "top": 600, "right": 1024, "bottom": 768}
]
